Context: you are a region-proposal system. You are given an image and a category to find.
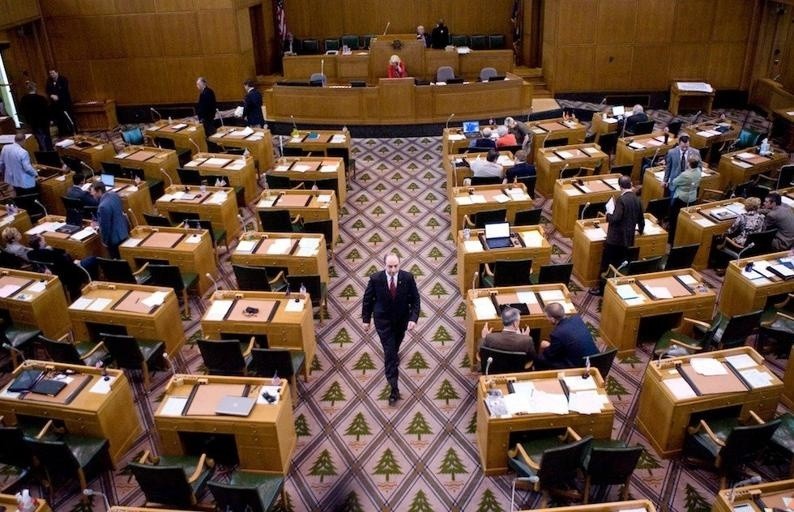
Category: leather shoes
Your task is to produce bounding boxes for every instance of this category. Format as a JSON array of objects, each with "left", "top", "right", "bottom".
[
  {"left": 589, "top": 288, "right": 601, "bottom": 295},
  {"left": 396, "top": 389, "right": 399, "bottom": 398},
  {"left": 389, "top": 392, "right": 395, "bottom": 406}
]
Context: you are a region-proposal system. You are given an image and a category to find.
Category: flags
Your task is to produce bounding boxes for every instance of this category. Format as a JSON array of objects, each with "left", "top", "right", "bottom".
[
  {"left": 275, "top": 0, "right": 287, "bottom": 41},
  {"left": 509, "top": 2, "right": 523, "bottom": 59}
]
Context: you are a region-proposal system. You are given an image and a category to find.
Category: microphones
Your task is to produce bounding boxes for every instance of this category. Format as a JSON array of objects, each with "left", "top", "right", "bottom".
[
  {"left": 2, "top": 343, "right": 26, "bottom": 362},
  {"left": 657, "top": 344, "right": 678, "bottom": 368},
  {"left": 263, "top": 172, "right": 269, "bottom": 190},
  {"left": 279, "top": 135, "right": 284, "bottom": 156},
  {"left": 690, "top": 111, "right": 702, "bottom": 124},
  {"left": 34, "top": 199, "right": 48, "bottom": 218},
  {"left": 82, "top": 488, "right": 111, "bottom": 512},
  {"left": 205, "top": 272, "right": 218, "bottom": 291},
  {"left": 237, "top": 214, "right": 246, "bottom": 233},
  {"left": 291, "top": 114, "right": 298, "bottom": 130},
  {"left": 472, "top": 271, "right": 479, "bottom": 294},
  {"left": 80, "top": 161, "right": 95, "bottom": 176},
  {"left": 559, "top": 163, "right": 569, "bottom": 180},
  {"left": 383, "top": 21, "right": 391, "bottom": 35},
  {"left": 727, "top": 138, "right": 741, "bottom": 152},
  {"left": 216, "top": 108, "right": 224, "bottom": 126},
  {"left": 446, "top": 113, "right": 455, "bottom": 128},
  {"left": 729, "top": 475, "right": 763, "bottom": 503},
  {"left": 160, "top": 167, "right": 173, "bottom": 186},
  {"left": 581, "top": 202, "right": 590, "bottom": 221},
  {"left": 485, "top": 356, "right": 494, "bottom": 380},
  {"left": 527, "top": 105, "right": 535, "bottom": 121},
  {"left": 128, "top": 208, "right": 139, "bottom": 227},
  {"left": 737, "top": 242, "right": 756, "bottom": 266},
  {"left": 162, "top": 352, "right": 176, "bottom": 375},
  {"left": 686, "top": 182, "right": 695, "bottom": 208},
  {"left": 614, "top": 260, "right": 629, "bottom": 284},
  {"left": 510, "top": 474, "right": 540, "bottom": 512},
  {"left": 598, "top": 98, "right": 607, "bottom": 107},
  {"left": 76, "top": 262, "right": 92, "bottom": 284},
  {"left": 150, "top": 107, "right": 162, "bottom": 121},
  {"left": 651, "top": 148, "right": 660, "bottom": 167},
  {"left": 321, "top": 59, "right": 324, "bottom": 75},
  {"left": 542, "top": 129, "right": 553, "bottom": 149},
  {"left": 189, "top": 137, "right": 201, "bottom": 154}
]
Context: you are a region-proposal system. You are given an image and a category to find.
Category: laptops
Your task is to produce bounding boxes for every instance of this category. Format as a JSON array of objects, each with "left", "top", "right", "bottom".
[
  {"left": 483, "top": 221, "right": 515, "bottom": 249},
  {"left": 55, "top": 208, "right": 84, "bottom": 234},
  {"left": 216, "top": 394, "right": 256, "bottom": 416},
  {"left": 612, "top": 104, "right": 626, "bottom": 120},
  {"left": 462, "top": 120, "right": 483, "bottom": 139},
  {"left": 100, "top": 173, "right": 115, "bottom": 191}
]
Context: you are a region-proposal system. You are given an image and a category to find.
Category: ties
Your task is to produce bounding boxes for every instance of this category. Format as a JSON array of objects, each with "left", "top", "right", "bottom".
[
  {"left": 681, "top": 151, "right": 685, "bottom": 170},
  {"left": 389, "top": 276, "right": 396, "bottom": 296}
]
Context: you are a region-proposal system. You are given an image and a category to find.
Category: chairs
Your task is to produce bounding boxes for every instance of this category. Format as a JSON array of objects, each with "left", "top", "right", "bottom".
[
  {"left": 725, "top": 180, "right": 754, "bottom": 199},
  {"left": 578, "top": 202, "right": 608, "bottom": 220},
  {"left": 324, "top": 39, "right": 339, "bottom": 55},
  {"left": 145, "top": 263, "right": 199, "bottom": 320},
  {"left": 602, "top": 255, "right": 662, "bottom": 282},
  {"left": 155, "top": 137, "right": 193, "bottom": 168},
  {"left": 513, "top": 175, "right": 537, "bottom": 202},
  {"left": 299, "top": 218, "right": 335, "bottom": 269},
  {"left": 283, "top": 146, "right": 313, "bottom": 158},
  {"left": 184, "top": 218, "right": 231, "bottom": 262},
  {"left": 583, "top": 347, "right": 618, "bottom": 383},
  {"left": 23, "top": 420, "right": 110, "bottom": 511},
  {"left": 584, "top": 439, "right": 644, "bottom": 505},
  {"left": 752, "top": 294, "right": 794, "bottom": 358},
  {"left": 38, "top": 331, "right": 107, "bottom": 368},
  {"left": 473, "top": 259, "right": 530, "bottom": 290},
  {"left": 93, "top": 257, "right": 151, "bottom": 288},
  {"left": 126, "top": 448, "right": 219, "bottom": 506},
  {"left": 288, "top": 274, "right": 329, "bottom": 322},
  {"left": 0, "top": 416, "right": 24, "bottom": 471},
  {"left": 197, "top": 335, "right": 257, "bottom": 377},
  {"left": 175, "top": 168, "right": 202, "bottom": 186},
  {"left": 462, "top": 176, "right": 502, "bottom": 186},
  {"left": 662, "top": 243, "right": 699, "bottom": 269},
  {"left": 715, "top": 228, "right": 778, "bottom": 274},
  {"left": 119, "top": 127, "right": 154, "bottom": 149},
  {"left": 60, "top": 196, "right": 87, "bottom": 227},
  {"left": 326, "top": 148, "right": 356, "bottom": 187},
  {"left": 99, "top": 332, "right": 173, "bottom": 394},
  {"left": 312, "top": 178, "right": 336, "bottom": 198},
  {"left": 680, "top": 410, "right": 780, "bottom": 490},
  {"left": 26, "top": 259, "right": 69, "bottom": 305},
  {"left": 436, "top": 66, "right": 455, "bottom": 82},
  {"left": 653, "top": 313, "right": 730, "bottom": 360},
  {"left": 618, "top": 121, "right": 654, "bottom": 139},
  {"left": 770, "top": 413, "right": 794, "bottom": 478},
  {"left": 530, "top": 262, "right": 573, "bottom": 287},
  {"left": 462, "top": 207, "right": 507, "bottom": 230},
  {"left": 265, "top": 174, "right": 306, "bottom": 190},
  {"left": 452, "top": 34, "right": 470, "bottom": 51},
  {"left": 642, "top": 198, "right": 673, "bottom": 232},
  {"left": 489, "top": 34, "right": 505, "bottom": 49},
  {"left": 255, "top": 208, "right": 305, "bottom": 236},
  {"left": 470, "top": 34, "right": 488, "bottom": 51},
  {"left": 207, "top": 470, "right": 288, "bottom": 512},
  {"left": 341, "top": 34, "right": 359, "bottom": 50},
  {"left": 734, "top": 128, "right": 768, "bottom": 151},
  {"left": 143, "top": 213, "right": 184, "bottom": 229},
  {"left": 708, "top": 139, "right": 733, "bottom": 171},
  {"left": 231, "top": 265, "right": 288, "bottom": 293},
  {"left": 251, "top": 346, "right": 308, "bottom": 407},
  {"left": 596, "top": 131, "right": 617, "bottom": 156},
  {"left": 302, "top": 38, "right": 318, "bottom": 55},
  {"left": 558, "top": 167, "right": 596, "bottom": 179},
  {"left": 480, "top": 68, "right": 497, "bottom": 83},
  {"left": 602, "top": 164, "right": 632, "bottom": 180},
  {"left": 311, "top": 73, "right": 326, "bottom": 86},
  {"left": 0, "top": 317, "right": 40, "bottom": 373},
  {"left": 753, "top": 165, "right": 794, "bottom": 200},
  {"left": 12, "top": 192, "right": 48, "bottom": 224},
  {"left": 510, "top": 207, "right": 541, "bottom": 226},
  {"left": 507, "top": 426, "right": 593, "bottom": 508},
  {"left": 479, "top": 339, "right": 534, "bottom": 376}
]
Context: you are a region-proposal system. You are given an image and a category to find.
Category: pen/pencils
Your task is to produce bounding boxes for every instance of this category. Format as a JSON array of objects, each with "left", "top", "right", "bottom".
[
  {"left": 250, "top": 384, "right": 259, "bottom": 393},
  {"left": 135, "top": 297, "right": 140, "bottom": 305},
  {"left": 530, "top": 389, "right": 534, "bottom": 399}
]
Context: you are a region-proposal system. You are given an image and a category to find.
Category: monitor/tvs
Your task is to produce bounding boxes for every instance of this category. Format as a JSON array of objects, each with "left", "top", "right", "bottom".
[
  {"left": 351, "top": 80, "right": 366, "bottom": 87},
  {"left": 309, "top": 80, "right": 322, "bottom": 87},
  {"left": 488, "top": 76, "right": 505, "bottom": 81},
  {"left": 446, "top": 78, "right": 464, "bottom": 84}
]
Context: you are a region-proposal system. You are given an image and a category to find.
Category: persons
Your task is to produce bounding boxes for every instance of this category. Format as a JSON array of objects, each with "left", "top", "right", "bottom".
[
  {"left": 480, "top": 308, "right": 537, "bottom": 365},
  {"left": 720, "top": 196, "right": 767, "bottom": 248},
  {"left": 25, "top": 232, "right": 81, "bottom": 302},
  {"left": 470, "top": 148, "right": 504, "bottom": 179},
  {"left": 661, "top": 133, "right": 701, "bottom": 203},
  {"left": 89, "top": 180, "right": 129, "bottom": 259},
  {"left": 387, "top": 54, "right": 409, "bottom": 78},
  {"left": 2, "top": 226, "right": 29, "bottom": 262},
  {"left": 616, "top": 105, "right": 649, "bottom": 140},
  {"left": 667, "top": 155, "right": 703, "bottom": 247},
  {"left": 194, "top": 76, "right": 221, "bottom": 155},
  {"left": 430, "top": 18, "right": 450, "bottom": 50},
  {"left": 541, "top": 301, "right": 600, "bottom": 371},
  {"left": 504, "top": 117, "right": 533, "bottom": 155},
  {"left": 18, "top": 79, "right": 58, "bottom": 169},
  {"left": 361, "top": 252, "right": 420, "bottom": 407},
  {"left": 237, "top": 79, "right": 267, "bottom": 129},
  {"left": 414, "top": 25, "right": 430, "bottom": 48},
  {"left": 495, "top": 125, "right": 518, "bottom": 149},
  {"left": 43, "top": 67, "right": 78, "bottom": 138},
  {"left": 0, "top": 132, "right": 42, "bottom": 223},
  {"left": 468, "top": 127, "right": 495, "bottom": 153},
  {"left": 504, "top": 149, "right": 537, "bottom": 200},
  {"left": 588, "top": 175, "right": 645, "bottom": 297},
  {"left": 762, "top": 193, "right": 794, "bottom": 252},
  {"left": 65, "top": 172, "right": 97, "bottom": 208}
]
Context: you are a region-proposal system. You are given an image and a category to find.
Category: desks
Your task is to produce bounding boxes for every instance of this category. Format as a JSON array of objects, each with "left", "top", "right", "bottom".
[
  {"left": 456, "top": 224, "right": 553, "bottom": 298},
  {"left": 206, "top": 125, "right": 275, "bottom": 174},
  {"left": 228, "top": 231, "right": 329, "bottom": 305},
  {"left": 202, "top": 289, "right": 318, "bottom": 383},
  {"left": 27, "top": 164, "right": 76, "bottom": 216},
  {"left": 1, "top": 266, "right": 70, "bottom": 344},
  {"left": 445, "top": 150, "right": 519, "bottom": 200},
  {"left": 282, "top": 129, "right": 350, "bottom": 178},
  {"left": 254, "top": 188, "right": 338, "bottom": 248},
  {"left": 534, "top": 142, "right": 610, "bottom": 200},
  {"left": 550, "top": 173, "right": 629, "bottom": 238},
  {"left": 80, "top": 175, "right": 153, "bottom": 228},
  {"left": 717, "top": 144, "right": 790, "bottom": 191},
  {"left": 0, "top": 204, "right": 30, "bottom": 255},
  {"left": 523, "top": 115, "right": 587, "bottom": 165},
  {"left": 117, "top": 224, "right": 220, "bottom": 294},
  {"left": 640, "top": 161, "right": 721, "bottom": 214},
  {"left": 0, "top": 359, "right": 142, "bottom": 470},
  {"left": 706, "top": 480, "right": 794, "bottom": 512},
  {"left": 449, "top": 182, "right": 536, "bottom": 246},
  {"left": 568, "top": 211, "right": 668, "bottom": 289},
  {"left": 599, "top": 267, "right": 719, "bottom": 356},
  {"left": 113, "top": 144, "right": 182, "bottom": 186},
  {"left": 143, "top": 119, "right": 209, "bottom": 158},
  {"left": 57, "top": 135, "right": 113, "bottom": 172},
  {"left": 614, "top": 131, "right": 678, "bottom": 186},
  {"left": 474, "top": 366, "right": 617, "bottom": 476},
  {"left": 153, "top": 374, "right": 297, "bottom": 480},
  {"left": 263, "top": 155, "right": 347, "bottom": 210},
  {"left": 443, "top": 124, "right": 512, "bottom": 165},
  {"left": 280, "top": 34, "right": 514, "bottom": 81},
  {"left": 671, "top": 197, "right": 762, "bottom": 273},
  {"left": 465, "top": 282, "right": 578, "bottom": 369},
  {"left": 156, "top": 183, "right": 239, "bottom": 250},
  {"left": 24, "top": 223, "right": 106, "bottom": 267},
  {"left": 717, "top": 248, "right": 793, "bottom": 324},
  {"left": 667, "top": 85, "right": 715, "bottom": 117},
  {"left": 67, "top": 280, "right": 188, "bottom": 366},
  {"left": 269, "top": 72, "right": 532, "bottom": 125},
  {"left": 683, "top": 117, "right": 746, "bottom": 162},
  {"left": 635, "top": 346, "right": 785, "bottom": 461},
  {"left": 182, "top": 150, "right": 256, "bottom": 205}
]
